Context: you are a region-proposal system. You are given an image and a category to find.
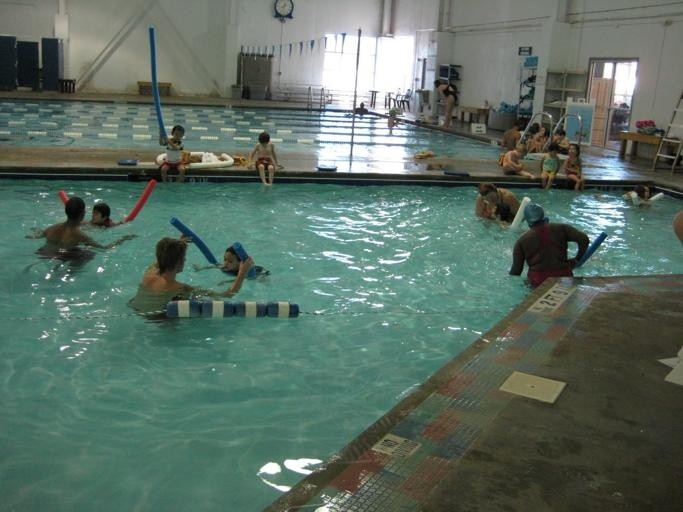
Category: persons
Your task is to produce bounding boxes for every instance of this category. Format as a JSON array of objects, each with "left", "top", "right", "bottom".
[
  {"left": 492, "top": 207, "right": 590, "bottom": 286},
  {"left": 491, "top": 202, "right": 512, "bottom": 229},
  {"left": 25, "top": 197, "right": 136, "bottom": 268},
  {"left": 674, "top": 210, "right": 683, "bottom": 244},
  {"left": 356, "top": 103, "right": 368, "bottom": 114},
  {"left": 128, "top": 233, "right": 253, "bottom": 316},
  {"left": 620, "top": 186, "right": 652, "bottom": 208},
  {"left": 161, "top": 124, "right": 186, "bottom": 182},
  {"left": 435, "top": 79, "right": 457, "bottom": 128},
  {"left": 251, "top": 129, "right": 284, "bottom": 189},
  {"left": 191, "top": 246, "right": 256, "bottom": 273},
  {"left": 502, "top": 121, "right": 585, "bottom": 192},
  {"left": 477, "top": 183, "right": 520, "bottom": 223},
  {"left": 87, "top": 202, "right": 123, "bottom": 228},
  {"left": 388, "top": 110, "right": 396, "bottom": 127}
]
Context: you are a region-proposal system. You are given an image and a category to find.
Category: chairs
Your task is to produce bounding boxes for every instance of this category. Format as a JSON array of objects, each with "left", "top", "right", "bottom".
[
  {"left": 385, "top": 87, "right": 401, "bottom": 109},
  {"left": 395, "top": 88, "right": 413, "bottom": 112}
]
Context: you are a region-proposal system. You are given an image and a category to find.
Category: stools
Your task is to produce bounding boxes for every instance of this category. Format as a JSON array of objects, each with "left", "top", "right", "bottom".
[{"left": 59, "top": 79, "right": 76, "bottom": 94}]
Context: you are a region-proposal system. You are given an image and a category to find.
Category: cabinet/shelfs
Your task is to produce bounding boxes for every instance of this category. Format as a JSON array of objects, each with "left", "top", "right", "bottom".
[
  {"left": 237, "top": 52, "right": 274, "bottom": 100},
  {"left": 432, "top": 63, "right": 463, "bottom": 120},
  {"left": 17, "top": 41, "right": 40, "bottom": 91},
  {"left": 517, "top": 67, "right": 538, "bottom": 120},
  {"left": 41, "top": 37, "right": 64, "bottom": 91},
  {"left": 541, "top": 68, "right": 588, "bottom": 130},
  {"left": 0, "top": 36, "right": 16, "bottom": 91}
]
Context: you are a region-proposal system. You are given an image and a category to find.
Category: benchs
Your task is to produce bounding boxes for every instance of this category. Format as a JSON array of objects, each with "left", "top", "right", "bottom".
[{"left": 276, "top": 84, "right": 332, "bottom": 104}]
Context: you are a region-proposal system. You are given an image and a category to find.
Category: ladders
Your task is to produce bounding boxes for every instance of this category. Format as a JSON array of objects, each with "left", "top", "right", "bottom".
[{"left": 652, "top": 91, "right": 683, "bottom": 175}]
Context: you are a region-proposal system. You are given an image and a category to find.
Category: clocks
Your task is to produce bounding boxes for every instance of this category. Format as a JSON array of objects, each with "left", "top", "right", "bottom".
[{"left": 274, "top": 0, "right": 294, "bottom": 18}]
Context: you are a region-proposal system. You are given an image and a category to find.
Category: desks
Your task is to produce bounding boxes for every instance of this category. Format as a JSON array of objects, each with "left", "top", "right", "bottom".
[
  {"left": 137, "top": 81, "right": 171, "bottom": 96},
  {"left": 460, "top": 106, "right": 490, "bottom": 127},
  {"left": 619, "top": 131, "right": 679, "bottom": 164}
]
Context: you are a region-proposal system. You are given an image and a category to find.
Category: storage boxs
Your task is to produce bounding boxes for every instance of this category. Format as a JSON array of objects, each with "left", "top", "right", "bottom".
[{"left": 488, "top": 108, "right": 516, "bottom": 131}]
